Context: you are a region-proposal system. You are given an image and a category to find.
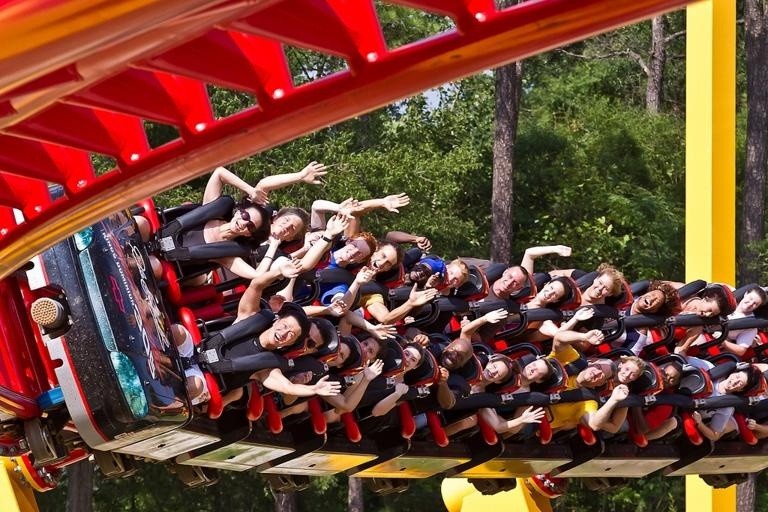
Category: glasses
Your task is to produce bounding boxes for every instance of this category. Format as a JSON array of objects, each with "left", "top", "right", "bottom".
[
  {"left": 305, "top": 332, "right": 316, "bottom": 349},
  {"left": 239, "top": 208, "right": 257, "bottom": 235}
]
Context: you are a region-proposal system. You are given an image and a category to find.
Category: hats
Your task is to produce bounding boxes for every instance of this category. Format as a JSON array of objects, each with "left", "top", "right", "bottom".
[{"left": 420, "top": 258, "right": 443, "bottom": 274}]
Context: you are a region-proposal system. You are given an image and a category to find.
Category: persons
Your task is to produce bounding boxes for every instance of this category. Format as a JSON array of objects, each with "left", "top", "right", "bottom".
[{"left": 130, "top": 160, "right": 768, "bottom": 448}]
